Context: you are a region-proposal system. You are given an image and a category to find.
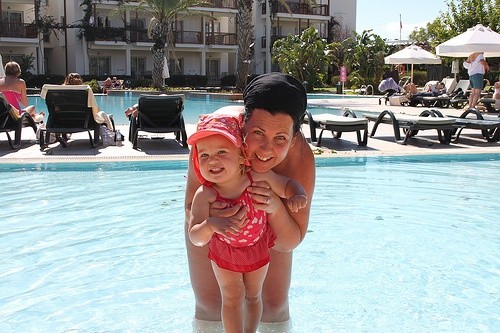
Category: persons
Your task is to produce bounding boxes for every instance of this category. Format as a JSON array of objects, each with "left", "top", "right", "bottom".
[
  {"left": 111, "top": 77, "right": 121, "bottom": 88},
  {"left": 185, "top": 72, "right": 315, "bottom": 322},
  {"left": 103, "top": 77, "right": 113, "bottom": 94},
  {"left": 405, "top": 82, "right": 445, "bottom": 106},
  {"left": 0, "top": 62, "right": 44, "bottom": 118},
  {"left": 463, "top": 52, "right": 489, "bottom": 109},
  {"left": 187, "top": 114, "right": 308, "bottom": 333},
  {"left": 62, "top": 73, "right": 82, "bottom": 85}
]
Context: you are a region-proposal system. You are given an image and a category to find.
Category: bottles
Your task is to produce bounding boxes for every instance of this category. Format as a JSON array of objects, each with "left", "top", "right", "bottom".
[
  {"left": 116, "top": 130, "right": 122, "bottom": 146},
  {"left": 103, "top": 129, "right": 108, "bottom": 147}
]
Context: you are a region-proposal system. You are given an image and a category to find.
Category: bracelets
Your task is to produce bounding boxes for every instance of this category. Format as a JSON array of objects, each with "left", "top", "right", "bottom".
[{"left": 301, "top": 195, "right": 307, "bottom": 204}]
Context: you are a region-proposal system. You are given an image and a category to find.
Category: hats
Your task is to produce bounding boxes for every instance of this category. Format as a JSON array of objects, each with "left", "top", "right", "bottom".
[
  {"left": 187, "top": 113, "right": 244, "bottom": 148},
  {"left": 244, "top": 71, "right": 307, "bottom": 121}
]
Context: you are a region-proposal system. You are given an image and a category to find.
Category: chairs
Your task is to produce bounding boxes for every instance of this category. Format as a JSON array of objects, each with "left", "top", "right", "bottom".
[
  {"left": 303, "top": 109, "right": 500, "bottom": 148},
  {"left": 39, "top": 84, "right": 117, "bottom": 149},
  {"left": 0, "top": 92, "right": 43, "bottom": 149},
  {"left": 130, "top": 94, "right": 188, "bottom": 149},
  {"left": 390, "top": 76, "right": 500, "bottom": 112}
]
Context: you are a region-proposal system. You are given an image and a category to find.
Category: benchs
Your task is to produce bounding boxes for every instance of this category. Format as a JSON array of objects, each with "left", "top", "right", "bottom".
[{"left": 97, "top": 80, "right": 124, "bottom": 93}]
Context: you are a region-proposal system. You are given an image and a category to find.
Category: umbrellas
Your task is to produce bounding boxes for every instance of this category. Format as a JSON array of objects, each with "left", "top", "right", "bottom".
[
  {"left": 385, "top": 43, "right": 442, "bottom": 82},
  {"left": 436, "top": 24, "right": 500, "bottom": 58}
]
